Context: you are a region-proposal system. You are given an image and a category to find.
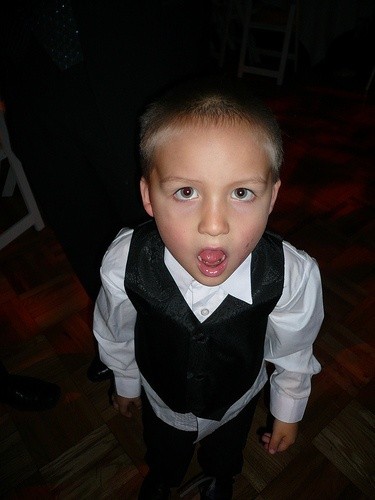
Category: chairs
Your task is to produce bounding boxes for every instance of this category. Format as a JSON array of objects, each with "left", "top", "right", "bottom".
[
  {"left": 0, "top": 111, "right": 45, "bottom": 251},
  {"left": 238, "top": 0, "right": 300, "bottom": 85}
]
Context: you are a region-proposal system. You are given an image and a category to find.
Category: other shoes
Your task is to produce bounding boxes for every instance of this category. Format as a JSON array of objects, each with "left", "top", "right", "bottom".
[
  {"left": 88, "top": 360, "right": 112, "bottom": 381},
  {"left": 0, "top": 375, "right": 59, "bottom": 408},
  {"left": 201, "top": 478, "right": 233, "bottom": 500},
  {"left": 140, "top": 487, "right": 172, "bottom": 500}
]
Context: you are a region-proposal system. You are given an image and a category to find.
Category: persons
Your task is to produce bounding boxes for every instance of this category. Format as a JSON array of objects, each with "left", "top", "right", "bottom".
[{"left": 93, "top": 80, "right": 325, "bottom": 500}]
